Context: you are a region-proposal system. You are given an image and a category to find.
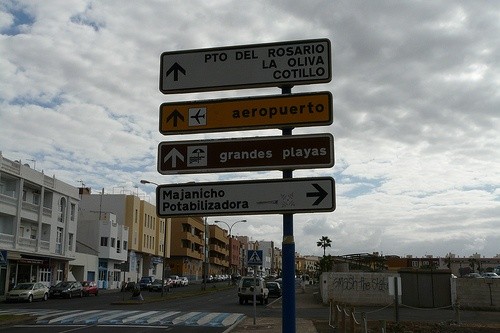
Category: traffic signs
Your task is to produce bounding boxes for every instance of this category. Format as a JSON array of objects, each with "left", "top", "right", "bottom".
[
  {"left": 155, "top": 176, "right": 336, "bottom": 218},
  {"left": 157, "top": 133, "right": 335, "bottom": 174},
  {"left": 158, "top": 91, "right": 336, "bottom": 136},
  {"left": 157, "top": 37, "right": 332, "bottom": 95}
]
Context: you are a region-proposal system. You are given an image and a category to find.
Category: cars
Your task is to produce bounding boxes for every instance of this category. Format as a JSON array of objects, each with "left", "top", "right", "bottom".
[
  {"left": 50, "top": 280, "right": 99, "bottom": 299},
  {"left": 139, "top": 275, "right": 189, "bottom": 293},
  {"left": 265, "top": 278, "right": 282, "bottom": 298},
  {"left": 6, "top": 282, "right": 49, "bottom": 303}
]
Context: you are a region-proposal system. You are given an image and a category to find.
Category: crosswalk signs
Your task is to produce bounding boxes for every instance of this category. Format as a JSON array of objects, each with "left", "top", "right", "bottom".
[{"left": 247, "top": 250, "right": 263, "bottom": 265}]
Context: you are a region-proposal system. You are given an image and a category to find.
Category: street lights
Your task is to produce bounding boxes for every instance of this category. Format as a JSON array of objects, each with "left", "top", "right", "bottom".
[
  {"left": 139, "top": 179, "right": 168, "bottom": 297},
  {"left": 214, "top": 220, "right": 247, "bottom": 287}
]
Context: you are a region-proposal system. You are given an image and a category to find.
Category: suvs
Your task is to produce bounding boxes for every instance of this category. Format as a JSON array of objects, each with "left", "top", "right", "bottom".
[{"left": 237, "top": 276, "right": 270, "bottom": 306}]
